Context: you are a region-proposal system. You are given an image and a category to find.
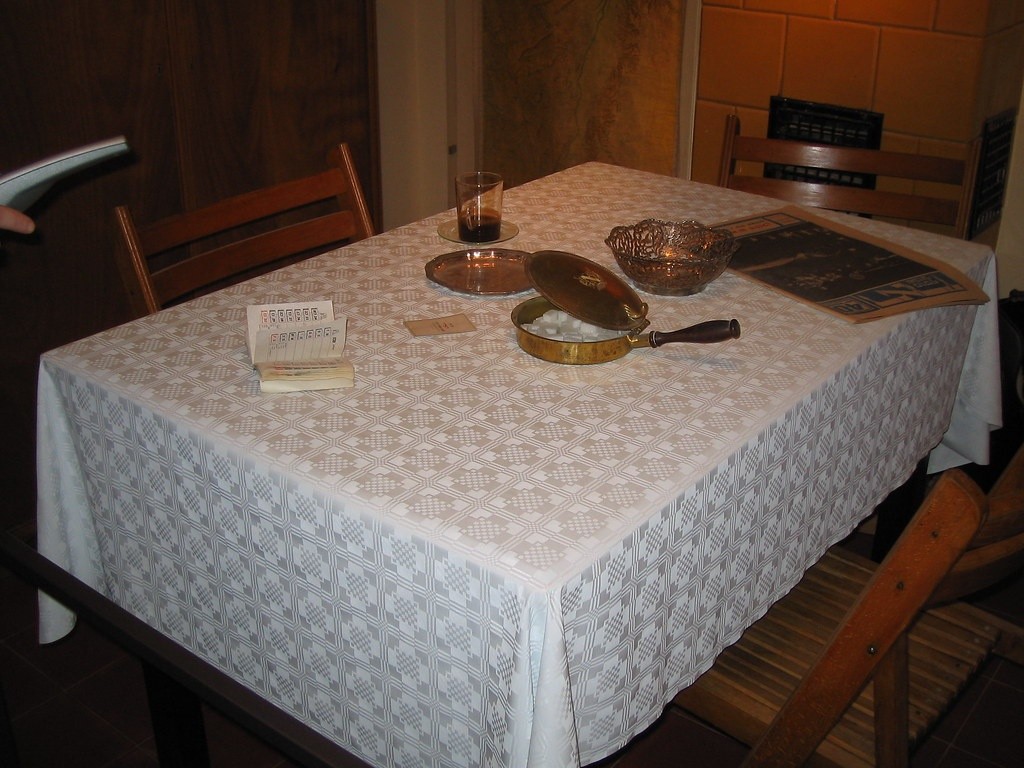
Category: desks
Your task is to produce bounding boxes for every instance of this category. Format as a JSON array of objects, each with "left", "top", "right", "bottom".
[{"left": 35, "top": 160, "right": 1005, "bottom": 768}]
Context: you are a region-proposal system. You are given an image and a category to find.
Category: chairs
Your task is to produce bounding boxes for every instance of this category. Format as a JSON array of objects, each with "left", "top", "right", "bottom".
[
  {"left": 718, "top": 112, "right": 984, "bottom": 241},
  {"left": 109, "top": 143, "right": 377, "bottom": 319},
  {"left": 670, "top": 443, "right": 1024, "bottom": 768}
]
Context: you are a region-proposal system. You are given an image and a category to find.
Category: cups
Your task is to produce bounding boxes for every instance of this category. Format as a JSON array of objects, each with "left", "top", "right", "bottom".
[{"left": 455, "top": 172, "right": 504, "bottom": 242}]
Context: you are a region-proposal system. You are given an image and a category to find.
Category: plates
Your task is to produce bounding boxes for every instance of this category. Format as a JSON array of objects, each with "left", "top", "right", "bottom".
[{"left": 425, "top": 249, "right": 533, "bottom": 296}]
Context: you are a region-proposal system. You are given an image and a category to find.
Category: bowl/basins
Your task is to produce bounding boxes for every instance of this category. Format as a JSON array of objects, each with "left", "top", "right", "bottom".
[{"left": 604, "top": 219, "right": 740, "bottom": 296}]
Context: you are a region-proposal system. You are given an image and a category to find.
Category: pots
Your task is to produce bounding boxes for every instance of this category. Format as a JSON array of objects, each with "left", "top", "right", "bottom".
[{"left": 511, "top": 249, "right": 740, "bottom": 365}]
